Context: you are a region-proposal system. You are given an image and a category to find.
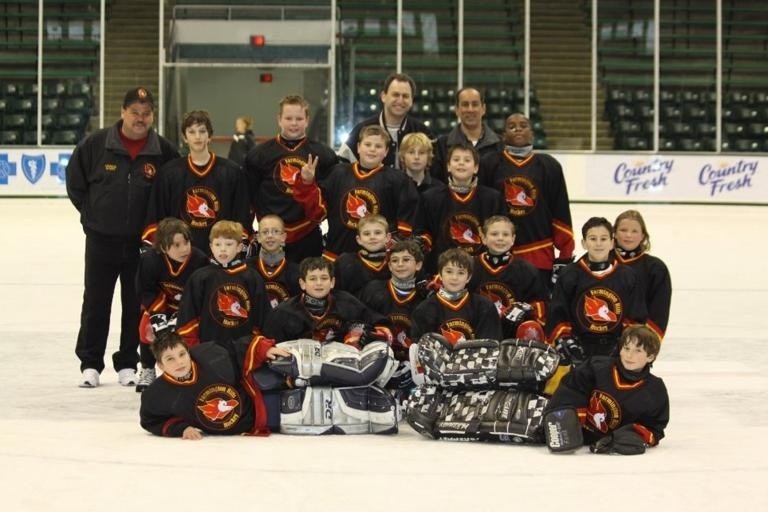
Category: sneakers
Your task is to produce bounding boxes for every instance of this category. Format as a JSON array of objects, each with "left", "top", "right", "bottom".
[{"left": 78, "top": 368, "right": 157, "bottom": 392}]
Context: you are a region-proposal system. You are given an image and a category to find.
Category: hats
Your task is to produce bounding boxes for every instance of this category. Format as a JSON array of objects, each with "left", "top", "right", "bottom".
[{"left": 125, "top": 87, "right": 154, "bottom": 110}]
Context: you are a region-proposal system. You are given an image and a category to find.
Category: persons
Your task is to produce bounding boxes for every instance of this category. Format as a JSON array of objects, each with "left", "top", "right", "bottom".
[
  {"left": 65, "top": 89, "right": 183, "bottom": 388},
  {"left": 136, "top": 75, "right": 671, "bottom": 391},
  {"left": 139, "top": 333, "right": 398, "bottom": 439},
  {"left": 408, "top": 325, "right": 669, "bottom": 448}
]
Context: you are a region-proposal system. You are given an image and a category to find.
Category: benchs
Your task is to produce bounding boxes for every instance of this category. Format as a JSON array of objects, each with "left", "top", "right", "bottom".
[
  {"left": 584, "top": 0, "right": 768, "bottom": 87},
  {"left": 335, "top": 0, "right": 524, "bottom": 86},
  {"left": 0, "top": 0, "right": 100, "bottom": 80}
]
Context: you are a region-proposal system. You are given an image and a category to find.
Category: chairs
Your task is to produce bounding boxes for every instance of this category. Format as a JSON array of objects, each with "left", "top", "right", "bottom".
[
  {"left": 0, "top": 77, "right": 92, "bottom": 146},
  {"left": 605, "top": 87, "right": 768, "bottom": 152},
  {"left": 342, "top": 84, "right": 547, "bottom": 149}
]
